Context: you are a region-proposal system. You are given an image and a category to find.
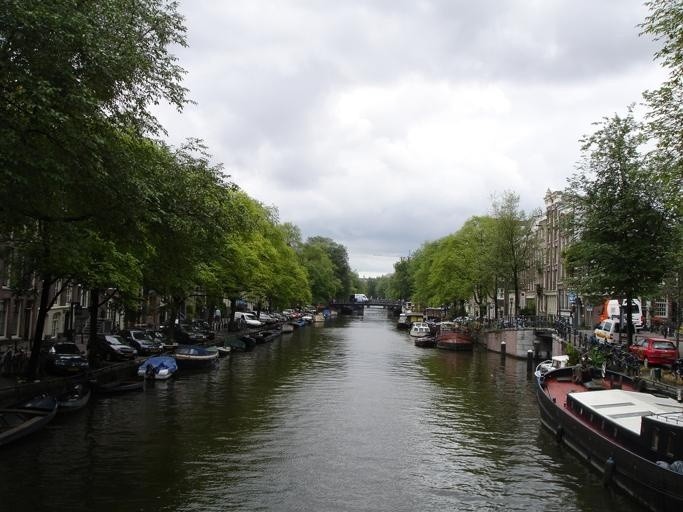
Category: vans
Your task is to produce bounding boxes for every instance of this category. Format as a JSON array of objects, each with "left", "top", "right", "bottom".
[{"left": 350, "top": 293, "right": 369, "bottom": 304}]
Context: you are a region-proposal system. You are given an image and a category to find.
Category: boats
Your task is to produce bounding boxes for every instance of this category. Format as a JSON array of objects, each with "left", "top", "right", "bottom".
[
  {"left": 533, "top": 351, "right": 683, "bottom": 511},
  {"left": 29, "top": 376, "right": 98, "bottom": 413},
  {"left": 100, "top": 341, "right": 233, "bottom": 397},
  {"left": 397, "top": 300, "right": 477, "bottom": 351},
  {"left": 233, "top": 302, "right": 338, "bottom": 350},
  {"left": 0, "top": 405, "right": 46, "bottom": 446}
]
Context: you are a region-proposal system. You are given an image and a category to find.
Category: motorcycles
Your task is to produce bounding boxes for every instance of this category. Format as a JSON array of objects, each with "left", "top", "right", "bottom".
[{"left": 660, "top": 317, "right": 679, "bottom": 339}]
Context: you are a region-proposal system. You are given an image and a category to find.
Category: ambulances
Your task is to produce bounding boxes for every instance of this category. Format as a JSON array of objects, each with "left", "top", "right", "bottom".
[{"left": 593, "top": 315, "right": 636, "bottom": 347}]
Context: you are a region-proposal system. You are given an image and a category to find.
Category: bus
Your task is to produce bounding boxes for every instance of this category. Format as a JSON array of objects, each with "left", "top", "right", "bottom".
[{"left": 593, "top": 297, "right": 644, "bottom": 333}]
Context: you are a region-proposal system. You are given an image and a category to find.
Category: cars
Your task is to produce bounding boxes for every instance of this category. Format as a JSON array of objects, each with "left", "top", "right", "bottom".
[
  {"left": 675, "top": 357, "right": 683, "bottom": 378},
  {"left": 629, "top": 336, "right": 679, "bottom": 369}
]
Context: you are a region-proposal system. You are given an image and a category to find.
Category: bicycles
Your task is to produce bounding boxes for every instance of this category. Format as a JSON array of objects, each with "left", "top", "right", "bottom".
[{"left": 1, "top": 344, "right": 28, "bottom": 374}]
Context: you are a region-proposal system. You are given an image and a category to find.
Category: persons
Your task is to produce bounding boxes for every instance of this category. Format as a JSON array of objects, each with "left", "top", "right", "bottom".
[
  {"left": 554, "top": 314, "right": 641, "bottom": 383},
  {"left": 464, "top": 312, "right": 555, "bottom": 332},
  {"left": 0, "top": 343, "right": 27, "bottom": 374}
]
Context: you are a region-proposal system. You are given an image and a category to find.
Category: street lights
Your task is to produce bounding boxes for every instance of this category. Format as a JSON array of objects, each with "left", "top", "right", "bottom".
[{"left": 508, "top": 296, "right": 513, "bottom": 328}]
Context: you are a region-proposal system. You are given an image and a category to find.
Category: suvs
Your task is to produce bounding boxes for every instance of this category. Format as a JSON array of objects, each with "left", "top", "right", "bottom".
[{"left": 46, "top": 318, "right": 217, "bottom": 374}]
